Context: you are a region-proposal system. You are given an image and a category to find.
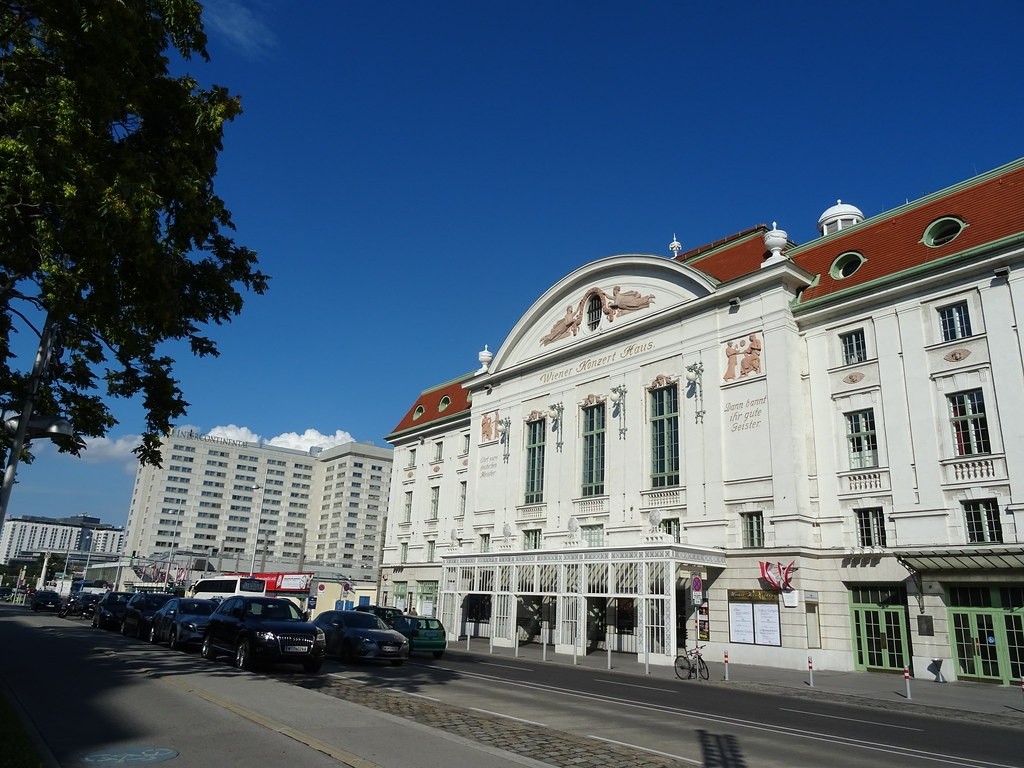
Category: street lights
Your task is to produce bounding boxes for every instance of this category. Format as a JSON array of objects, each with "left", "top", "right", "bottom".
[
  {"left": 249, "top": 481, "right": 267, "bottom": 576},
  {"left": 12, "top": 566, "right": 27, "bottom": 606},
  {"left": 79, "top": 535, "right": 97, "bottom": 591},
  {"left": 163, "top": 509, "right": 179, "bottom": 592}
]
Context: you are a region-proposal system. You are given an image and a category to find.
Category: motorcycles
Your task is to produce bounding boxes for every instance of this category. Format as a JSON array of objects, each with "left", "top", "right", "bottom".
[{"left": 58, "top": 594, "right": 96, "bottom": 620}]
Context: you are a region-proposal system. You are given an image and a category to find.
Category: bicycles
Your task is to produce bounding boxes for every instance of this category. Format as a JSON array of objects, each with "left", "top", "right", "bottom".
[{"left": 674, "top": 644, "right": 710, "bottom": 681}]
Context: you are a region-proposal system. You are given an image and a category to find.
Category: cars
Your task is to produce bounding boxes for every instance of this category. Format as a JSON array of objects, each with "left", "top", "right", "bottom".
[
  {"left": 54, "top": 572, "right": 66, "bottom": 579},
  {"left": 30, "top": 590, "right": 63, "bottom": 614},
  {"left": 0, "top": 586, "right": 12, "bottom": 597},
  {"left": 121, "top": 592, "right": 177, "bottom": 641},
  {"left": 313, "top": 609, "right": 410, "bottom": 665},
  {"left": 201, "top": 595, "right": 327, "bottom": 670},
  {"left": 385, "top": 616, "right": 447, "bottom": 658},
  {"left": 91, "top": 592, "right": 136, "bottom": 630},
  {"left": 44, "top": 580, "right": 58, "bottom": 591},
  {"left": 349, "top": 605, "right": 404, "bottom": 622},
  {"left": 147, "top": 597, "right": 221, "bottom": 651}
]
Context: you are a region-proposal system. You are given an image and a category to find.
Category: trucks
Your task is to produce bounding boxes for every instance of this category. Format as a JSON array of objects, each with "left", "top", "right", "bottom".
[{"left": 182, "top": 571, "right": 311, "bottom": 620}]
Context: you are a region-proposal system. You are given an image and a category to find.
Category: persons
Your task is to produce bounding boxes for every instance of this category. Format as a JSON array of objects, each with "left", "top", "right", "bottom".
[
  {"left": 603, "top": 286, "right": 656, "bottom": 311},
  {"left": 722, "top": 333, "right": 762, "bottom": 383},
  {"left": 481, "top": 409, "right": 500, "bottom": 441},
  {"left": 654, "top": 374, "right": 666, "bottom": 387},
  {"left": 403, "top": 608, "right": 408, "bottom": 615},
  {"left": 410, "top": 607, "right": 418, "bottom": 616},
  {"left": 530, "top": 411, "right": 538, "bottom": 420},
  {"left": 587, "top": 393, "right": 594, "bottom": 404},
  {"left": 539, "top": 299, "right": 582, "bottom": 347}
]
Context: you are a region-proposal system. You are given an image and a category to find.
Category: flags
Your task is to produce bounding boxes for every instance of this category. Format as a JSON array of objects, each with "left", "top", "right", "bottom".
[{"left": 760, "top": 562, "right": 796, "bottom": 592}]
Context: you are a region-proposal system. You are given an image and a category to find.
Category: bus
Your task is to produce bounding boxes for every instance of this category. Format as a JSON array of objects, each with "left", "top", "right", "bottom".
[{"left": 71, "top": 580, "right": 93, "bottom": 594}]
